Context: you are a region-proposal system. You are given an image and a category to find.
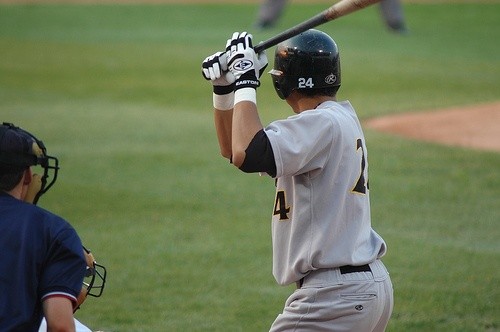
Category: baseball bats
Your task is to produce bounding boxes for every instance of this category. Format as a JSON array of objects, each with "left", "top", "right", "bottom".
[{"left": 253, "top": 0, "right": 382, "bottom": 51}]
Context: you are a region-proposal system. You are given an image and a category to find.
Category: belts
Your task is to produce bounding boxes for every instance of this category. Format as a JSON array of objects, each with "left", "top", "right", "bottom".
[{"left": 299, "top": 264, "right": 372, "bottom": 288}]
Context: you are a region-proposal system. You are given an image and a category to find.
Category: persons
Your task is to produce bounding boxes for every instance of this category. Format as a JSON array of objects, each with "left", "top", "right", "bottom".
[
  {"left": 256, "top": 0, "right": 407, "bottom": 34},
  {"left": 201, "top": 30, "right": 394, "bottom": 332},
  {"left": 0, "top": 121, "right": 107, "bottom": 332}
]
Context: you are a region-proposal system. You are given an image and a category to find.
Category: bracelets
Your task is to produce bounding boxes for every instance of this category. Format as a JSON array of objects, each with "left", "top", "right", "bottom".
[
  {"left": 212, "top": 91, "right": 234, "bottom": 110},
  {"left": 234, "top": 87, "right": 256, "bottom": 104}
]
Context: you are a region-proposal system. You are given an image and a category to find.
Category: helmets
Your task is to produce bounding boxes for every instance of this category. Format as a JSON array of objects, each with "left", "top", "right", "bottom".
[
  {"left": 269, "top": 28, "right": 342, "bottom": 100},
  {"left": 0, "top": 121, "right": 47, "bottom": 168}
]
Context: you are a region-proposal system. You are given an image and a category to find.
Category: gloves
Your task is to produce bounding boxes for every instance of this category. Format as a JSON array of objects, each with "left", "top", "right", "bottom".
[
  {"left": 225, "top": 30, "right": 269, "bottom": 93},
  {"left": 201, "top": 51, "right": 236, "bottom": 95}
]
